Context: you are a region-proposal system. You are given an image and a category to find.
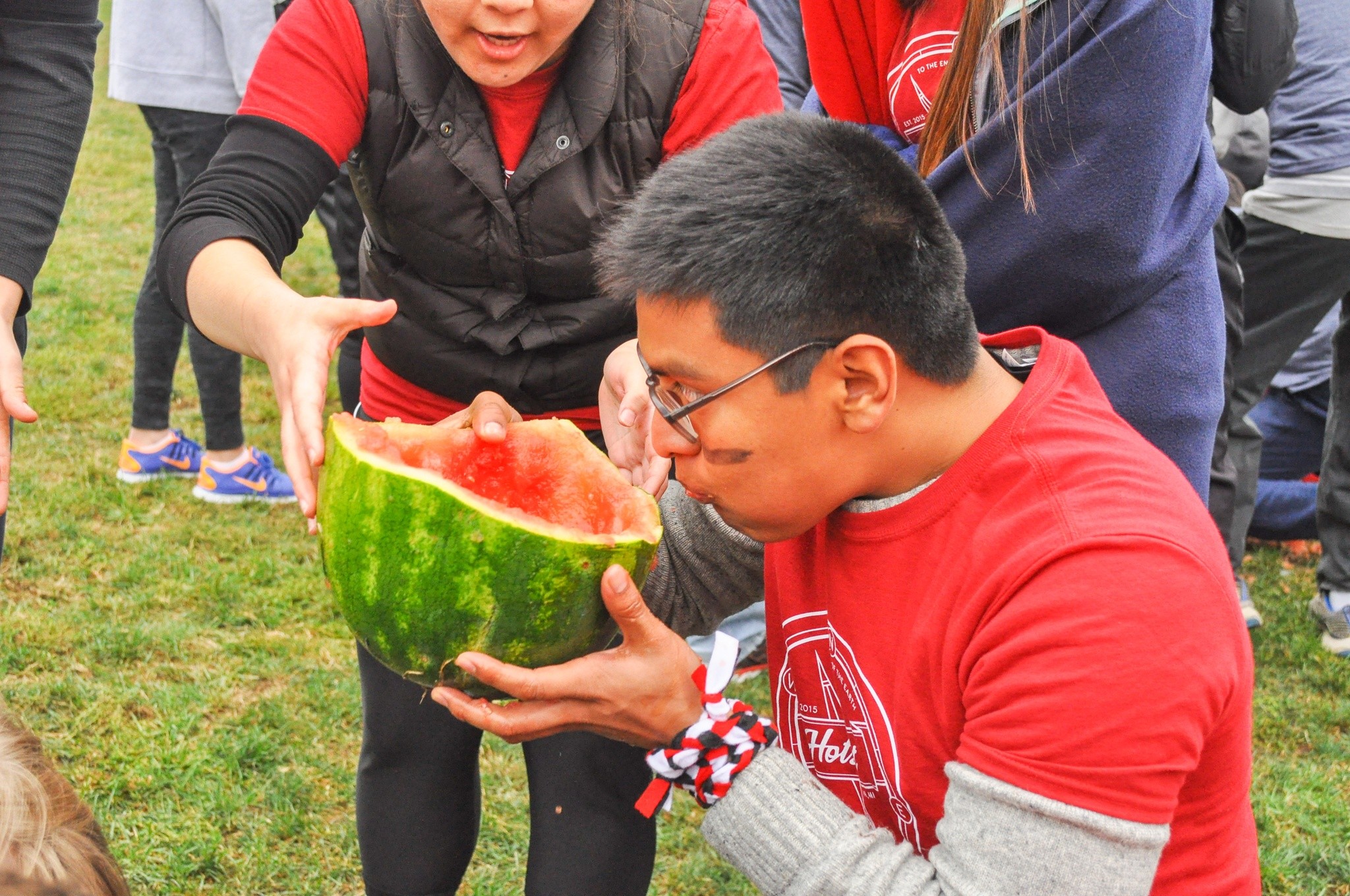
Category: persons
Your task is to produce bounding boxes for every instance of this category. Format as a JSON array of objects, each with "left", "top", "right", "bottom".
[
  {"left": 154, "top": 0, "right": 787, "bottom": 896},
  {"left": 431, "top": 111, "right": 1262, "bottom": 896},
  {"left": 1, "top": 0, "right": 1349, "bottom": 660}
]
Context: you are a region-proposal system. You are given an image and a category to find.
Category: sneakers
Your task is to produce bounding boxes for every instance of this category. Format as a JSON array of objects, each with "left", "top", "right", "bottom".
[
  {"left": 116, "top": 428, "right": 204, "bottom": 484},
  {"left": 192, "top": 446, "right": 297, "bottom": 505}
]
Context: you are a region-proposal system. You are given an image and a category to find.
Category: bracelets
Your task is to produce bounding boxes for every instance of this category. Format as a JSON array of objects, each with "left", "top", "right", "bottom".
[{"left": 636, "top": 629, "right": 778, "bottom": 818}]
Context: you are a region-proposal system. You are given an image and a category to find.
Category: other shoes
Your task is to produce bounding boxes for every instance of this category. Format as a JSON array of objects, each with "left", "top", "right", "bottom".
[
  {"left": 1237, "top": 580, "right": 1263, "bottom": 630},
  {"left": 1307, "top": 592, "right": 1350, "bottom": 658}
]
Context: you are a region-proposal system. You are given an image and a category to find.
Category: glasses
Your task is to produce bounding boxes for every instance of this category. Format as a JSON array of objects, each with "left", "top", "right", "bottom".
[{"left": 636, "top": 343, "right": 830, "bottom": 444}]
